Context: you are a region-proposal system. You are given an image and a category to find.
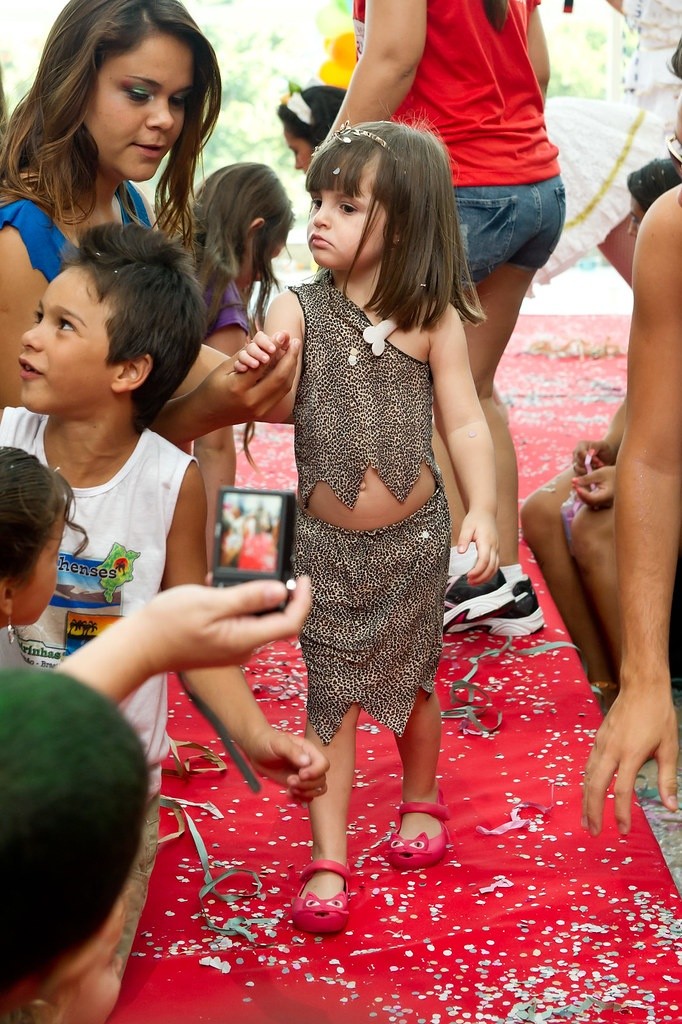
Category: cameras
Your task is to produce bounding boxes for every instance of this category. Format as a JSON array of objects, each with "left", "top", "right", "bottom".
[{"left": 212, "top": 484, "right": 296, "bottom": 614}]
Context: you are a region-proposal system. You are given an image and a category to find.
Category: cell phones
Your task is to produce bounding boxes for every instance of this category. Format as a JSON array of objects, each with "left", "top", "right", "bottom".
[{"left": 585, "top": 452, "right": 600, "bottom": 510}]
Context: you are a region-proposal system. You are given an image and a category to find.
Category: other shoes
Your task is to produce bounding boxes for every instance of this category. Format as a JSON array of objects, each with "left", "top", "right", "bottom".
[
  {"left": 592, "top": 682, "right": 617, "bottom": 713},
  {"left": 387, "top": 790, "right": 446, "bottom": 870},
  {"left": 291, "top": 855, "right": 349, "bottom": 932}
]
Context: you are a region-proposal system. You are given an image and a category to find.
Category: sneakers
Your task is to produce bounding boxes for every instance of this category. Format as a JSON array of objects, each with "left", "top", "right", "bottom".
[{"left": 440, "top": 567, "right": 545, "bottom": 638}]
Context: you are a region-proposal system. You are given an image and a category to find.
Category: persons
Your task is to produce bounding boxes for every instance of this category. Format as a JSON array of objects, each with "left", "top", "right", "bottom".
[
  {"left": 520, "top": 159, "right": 682, "bottom": 716},
  {"left": 220, "top": 499, "right": 281, "bottom": 571},
  {"left": 0, "top": 667, "right": 150, "bottom": 1024},
  {"left": 232, "top": 102, "right": 501, "bottom": 935},
  {"left": 0, "top": 223, "right": 331, "bottom": 977},
  {"left": 607, "top": 0, "right": 682, "bottom": 122},
  {"left": 0, "top": 889, "right": 124, "bottom": 1024},
  {"left": 278, "top": 85, "right": 663, "bottom": 298},
  {"left": 0, "top": 0, "right": 306, "bottom": 447},
  {"left": 181, "top": 161, "right": 301, "bottom": 585},
  {"left": 312, "top": 0, "right": 566, "bottom": 637},
  {"left": 0, "top": 447, "right": 89, "bottom": 630},
  {"left": 584, "top": 31, "right": 682, "bottom": 836}
]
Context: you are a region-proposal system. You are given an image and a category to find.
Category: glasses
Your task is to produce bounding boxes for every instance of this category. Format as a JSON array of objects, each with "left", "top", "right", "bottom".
[{"left": 668, "top": 136, "right": 682, "bottom": 179}]
